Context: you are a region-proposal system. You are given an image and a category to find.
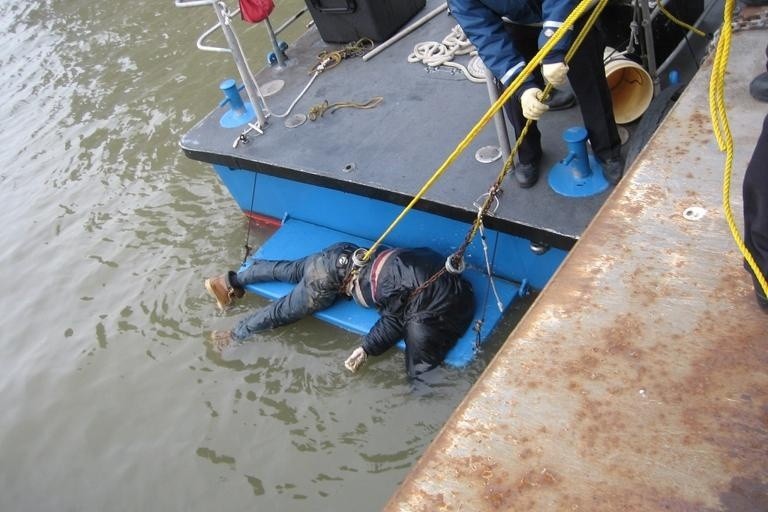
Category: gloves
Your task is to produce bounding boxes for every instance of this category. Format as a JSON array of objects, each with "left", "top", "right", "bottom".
[
  {"left": 543, "top": 62, "right": 570, "bottom": 85},
  {"left": 345, "top": 347, "right": 367, "bottom": 374},
  {"left": 520, "top": 87, "right": 549, "bottom": 120}
]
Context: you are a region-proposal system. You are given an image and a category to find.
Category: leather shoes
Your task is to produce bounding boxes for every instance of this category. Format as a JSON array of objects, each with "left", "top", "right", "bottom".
[
  {"left": 744, "top": 258, "right": 768, "bottom": 306},
  {"left": 595, "top": 154, "right": 625, "bottom": 184},
  {"left": 515, "top": 161, "right": 539, "bottom": 188},
  {"left": 749, "top": 72, "right": 768, "bottom": 102}
]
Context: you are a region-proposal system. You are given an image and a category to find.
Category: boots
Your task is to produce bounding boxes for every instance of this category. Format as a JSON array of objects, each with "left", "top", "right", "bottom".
[
  {"left": 210, "top": 329, "right": 235, "bottom": 352},
  {"left": 205, "top": 270, "right": 245, "bottom": 309}
]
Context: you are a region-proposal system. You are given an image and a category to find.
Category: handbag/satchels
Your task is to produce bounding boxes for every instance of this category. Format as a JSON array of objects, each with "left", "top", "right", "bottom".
[{"left": 239, "top": 0, "right": 275, "bottom": 24}]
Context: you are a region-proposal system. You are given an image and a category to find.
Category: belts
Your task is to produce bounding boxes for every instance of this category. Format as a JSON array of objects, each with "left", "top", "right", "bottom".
[{"left": 336, "top": 247, "right": 357, "bottom": 281}]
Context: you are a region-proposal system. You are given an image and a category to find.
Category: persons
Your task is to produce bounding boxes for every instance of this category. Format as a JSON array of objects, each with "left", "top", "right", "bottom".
[
  {"left": 203, "top": 243, "right": 472, "bottom": 375},
  {"left": 449, "top": 0, "right": 625, "bottom": 188}
]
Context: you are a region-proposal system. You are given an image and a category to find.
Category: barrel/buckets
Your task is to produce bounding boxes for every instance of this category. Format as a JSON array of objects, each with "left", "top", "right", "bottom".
[{"left": 602, "top": 47, "right": 654, "bottom": 124}]
